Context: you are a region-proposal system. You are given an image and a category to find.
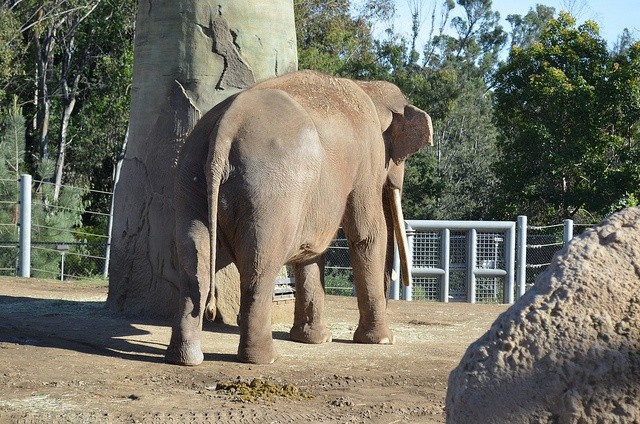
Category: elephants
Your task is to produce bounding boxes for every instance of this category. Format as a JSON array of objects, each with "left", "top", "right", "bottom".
[{"left": 164, "top": 68, "right": 434, "bottom": 366}]
